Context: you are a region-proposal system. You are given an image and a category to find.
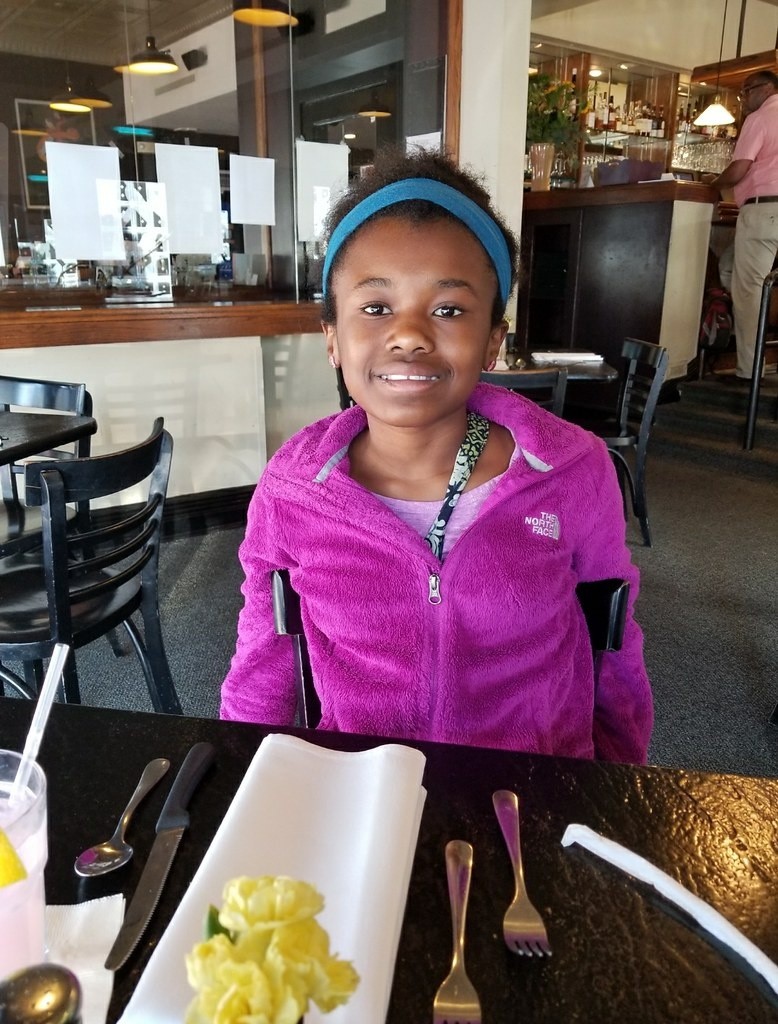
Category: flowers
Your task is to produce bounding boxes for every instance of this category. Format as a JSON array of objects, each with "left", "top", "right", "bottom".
[
  {"left": 524, "top": 73, "right": 592, "bottom": 167},
  {"left": 182, "top": 877, "right": 359, "bottom": 1024}
]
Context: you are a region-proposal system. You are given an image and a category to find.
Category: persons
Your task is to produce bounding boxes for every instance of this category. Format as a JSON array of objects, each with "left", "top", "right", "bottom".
[
  {"left": 220, "top": 147, "right": 654, "bottom": 766},
  {"left": 701, "top": 70, "right": 778, "bottom": 386}
]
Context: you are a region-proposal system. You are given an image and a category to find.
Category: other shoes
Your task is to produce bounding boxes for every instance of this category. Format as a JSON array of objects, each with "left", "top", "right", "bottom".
[{"left": 733, "top": 373, "right": 764, "bottom": 387}]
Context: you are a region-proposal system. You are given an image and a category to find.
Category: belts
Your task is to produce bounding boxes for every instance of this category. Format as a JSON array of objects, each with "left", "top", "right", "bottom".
[{"left": 743, "top": 195, "right": 778, "bottom": 205}]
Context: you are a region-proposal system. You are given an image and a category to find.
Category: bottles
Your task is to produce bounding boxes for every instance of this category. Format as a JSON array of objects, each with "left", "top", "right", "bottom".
[
  {"left": 586, "top": 91, "right": 666, "bottom": 140},
  {"left": 0, "top": 962, "right": 85, "bottom": 1024},
  {"left": 676, "top": 99, "right": 699, "bottom": 135}
]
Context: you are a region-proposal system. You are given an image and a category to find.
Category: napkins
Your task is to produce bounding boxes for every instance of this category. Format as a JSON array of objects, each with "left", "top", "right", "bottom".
[
  {"left": 531, "top": 352, "right": 604, "bottom": 362},
  {"left": 45, "top": 893, "right": 125, "bottom": 1024},
  {"left": 119, "top": 733, "right": 429, "bottom": 1024}
]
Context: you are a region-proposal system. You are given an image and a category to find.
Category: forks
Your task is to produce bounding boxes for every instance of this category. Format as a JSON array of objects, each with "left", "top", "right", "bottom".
[
  {"left": 432, "top": 839, "right": 483, "bottom": 1024},
  {"left": 492, "top": 790, "right": 553, "bottom": 958}
]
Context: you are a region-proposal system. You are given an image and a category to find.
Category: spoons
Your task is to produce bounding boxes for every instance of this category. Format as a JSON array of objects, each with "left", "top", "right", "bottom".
[{"left": 74, "top": 757, "right": 172, "bottom": 877}]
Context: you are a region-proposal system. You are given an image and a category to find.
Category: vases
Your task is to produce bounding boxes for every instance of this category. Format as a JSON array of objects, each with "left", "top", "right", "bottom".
[{"left": 530, "top": 142, "right": 556, "bottom": 192}]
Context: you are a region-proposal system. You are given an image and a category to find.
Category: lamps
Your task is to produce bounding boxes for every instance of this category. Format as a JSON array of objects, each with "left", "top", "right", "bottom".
[
  {"left": 360, "top": 91, "right": 392, "bottom": 119},
  {"left": 48, "top": 0, "right": 178, "bottom": 111},
  {"left": 232, "top": 0, "right": 299, "bottom": 28},
  {"left": 694, "top": 0, "right": 736, "bottom": 126}
]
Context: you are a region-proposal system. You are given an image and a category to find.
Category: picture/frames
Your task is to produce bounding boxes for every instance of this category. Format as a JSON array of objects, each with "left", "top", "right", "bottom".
[
  {"left": 7, "top": 93, "right": 101, "bottom": 213},
  {"left": 0, "top": 0, "right": 466, "bottom": 350}
]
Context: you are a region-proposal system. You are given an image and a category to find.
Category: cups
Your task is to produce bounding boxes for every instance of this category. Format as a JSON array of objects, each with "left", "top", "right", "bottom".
[
  {"left": 0, "top": 748, "right": 51, "bottom": 984},
  {"left": 671, "top": 140, "right": 736, "bottom": 175}
]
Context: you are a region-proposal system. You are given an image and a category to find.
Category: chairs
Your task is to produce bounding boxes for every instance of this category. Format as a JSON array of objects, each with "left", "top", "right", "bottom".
[{"left": 0, "top": 337, "right": 672, "bottom": 759}]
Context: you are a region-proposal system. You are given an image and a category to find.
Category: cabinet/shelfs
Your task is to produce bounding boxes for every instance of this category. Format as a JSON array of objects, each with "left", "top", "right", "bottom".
[{"left": 528, "top": 42, "right": 778, "bottom": 182}]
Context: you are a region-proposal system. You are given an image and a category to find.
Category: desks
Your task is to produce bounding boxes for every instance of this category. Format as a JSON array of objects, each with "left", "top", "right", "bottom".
[
  {"left": 0, "top": 408, "right": 98, "bottom": 466},
  {"left": 0, "top": 698, "right": 778, "bottom": 1024},
  {"left": 491, "top": 346, "right": 618, "bottom": 383}
]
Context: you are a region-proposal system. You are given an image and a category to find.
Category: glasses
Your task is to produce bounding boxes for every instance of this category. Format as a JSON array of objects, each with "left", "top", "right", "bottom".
[{"left": 737, "top": 80, "right": 770, "bottom": 102}]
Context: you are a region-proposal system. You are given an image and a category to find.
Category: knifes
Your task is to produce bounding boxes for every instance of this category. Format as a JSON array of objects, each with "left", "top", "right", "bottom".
[{"left": 103, "top": 742, "right": 213, "bottom": 972}]
